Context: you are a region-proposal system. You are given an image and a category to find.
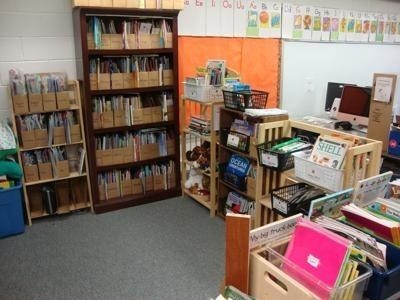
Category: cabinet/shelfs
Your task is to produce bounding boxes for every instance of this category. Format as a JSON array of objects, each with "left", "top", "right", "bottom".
[
  {"left": 7, "top": 82, "right": 95, "bottom": 228},
  {"left": 211, "top": 105, "right": 286, "bottom": 222},
  {"left": 72, "top": 6, "right": 187, "bottom": 215},
  {"left": 253, "top": 120, "right": 382, "bottom": 233},
  {"left": 181, "top": 98, "right": 227, "bottom": 215}
]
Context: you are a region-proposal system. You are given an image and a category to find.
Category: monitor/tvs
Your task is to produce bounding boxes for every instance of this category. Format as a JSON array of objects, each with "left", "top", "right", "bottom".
[
  {"left": 336, "top": 85, "right": 372, "bottom": 133},
  {"left": 325, "top": 82, "right": 355, "bottom": 119}
]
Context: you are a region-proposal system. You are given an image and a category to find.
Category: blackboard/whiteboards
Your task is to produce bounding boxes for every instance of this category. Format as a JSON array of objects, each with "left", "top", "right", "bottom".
[{"left": 279, "top": 39, "right": 400, "bottom": 122}]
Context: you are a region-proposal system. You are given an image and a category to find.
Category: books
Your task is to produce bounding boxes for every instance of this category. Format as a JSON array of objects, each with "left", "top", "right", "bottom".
[
  {"left": 10, "top": 72, "right": 83, "bottom": 177},
  {"left": 309, "top": 132, "right": 363, "bottom": 170},
  {"left": 272, "top": 137, "right": 309, "bottom": 153},
  {"left": 84, "top": 13, "right": 178, "bottom": 198},
  {"left": 221, "top": 117, "right": 255, "bottom": 217},
  {"left": 281, "top": 176, "right": 400, "bottom": 300},
  {"left": 185, "top": 60, "right": 226, "bottom": 98},
  {"left": 224, "top": 283, "right": 254, "bottom": 300},
  {"left": 245, "top": 108, "right": 289, "bottom": 123},
  {"left": 188, "top": 116, "right": 213, "bottom": 134}
]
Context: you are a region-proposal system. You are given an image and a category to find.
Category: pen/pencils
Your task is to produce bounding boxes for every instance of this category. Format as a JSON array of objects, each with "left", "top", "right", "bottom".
[{"left": 208, "top": 67, "right": 222, "bottom": 85}]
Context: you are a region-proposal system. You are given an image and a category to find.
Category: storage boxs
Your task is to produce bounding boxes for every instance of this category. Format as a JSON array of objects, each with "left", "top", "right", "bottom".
[
  {"left": 28, "top": 94, "right": 43, "bottom": 112},
  {"left": 52, "top": 127, "right": 66, "bottom": 144},
  {"left": 140, "top": 70, "right": 159, "bottom": 86},
  {"left": 86, "top": 34, "right": 123, "bottom": 49},
  {"left": 291, "top": 150, "right": 370, "bottom": 194},
  {"left": 19, "top": 117, "right": 49, "bottom": 148},
  {"left": 166, "top": 141, "right": 173, "bottom": 155},
  {"left": 220, "top": 108, "right": 288, "bottom": 152},
  {"left": 145, "top": 176, "right": 162, "bottom": 192},
  {"left": 163, "top": 70, "right": 174, "bottom": 86},
  {"left": 55, "top": 92, "right": 70, "bottom": 109},
  {"left": 57, "top": 161, "right": 70, "bottom": 178},
  {"left": 139, "top": 142, "right": 161, "bottom": 160},
  {"left": 123, "top": 179, "right": 141, "bottom": 197},
  {"left": 219, "top": 165, "right": 257, "bottom": 194},
  {"left": 99, "top": 185, "right": 120, "bottom": 199},
  {"left": 168, "top": 174, "right": 176, "bottom": 189},
  {"left": 24, "top": 164, "right": 37, "bottom": 182},
  {"left": 218, "top": 200, "right": 255, "bottom": 223},
  {"left": 11, "top": 83, "right": 28, "bottom": 113},
  {"left": 167, "top": 99, "right": 174, "bottom": 121},
  {"left": 42, "top": 92, "right": 57, "bottom": 110},
  {"left": 90, "top": 72, "right": 135, "bottom": 89},
  {"left": 70, "top": 125, "right": 81, "bottom": 142},
  {"left": 92, "top": 111, "right": 125, "bottom": 129},
  {"left": 366, "top": 73, "right": 397, "bottom": 153},
  {"left": 39, "top": 161, "right": 52, "bottom": 180},
  {"left": 95, "top": 146, "right": 132, "bottom": 165},
  {"left": 131, "top": 106, "right": 161, "bottom": 125},
  {"left": 127, "top": 32, "right": 172, "bottom": 49}
]
